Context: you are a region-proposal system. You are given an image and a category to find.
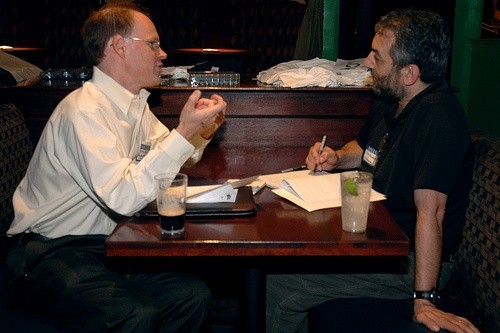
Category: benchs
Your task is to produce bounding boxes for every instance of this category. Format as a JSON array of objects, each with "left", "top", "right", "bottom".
[
  {"left": 0, "top": 103, "right": 33, "bottom": 243},
  {"left": 307, "top": 128, "right": 500, "bottom": 333}
]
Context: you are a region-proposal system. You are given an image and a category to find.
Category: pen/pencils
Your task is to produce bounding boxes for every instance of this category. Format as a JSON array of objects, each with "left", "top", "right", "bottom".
[
  {"left": 313, "top": 136, "right": 326, "bottom": 175},
  {"left": 280, "top": 165, "right": 306, "bottom": 173}
]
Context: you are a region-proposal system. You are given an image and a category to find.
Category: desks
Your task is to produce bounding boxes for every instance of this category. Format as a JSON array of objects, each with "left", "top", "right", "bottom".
[{"left": 103, "top": 182, "right": 411, "bottom": 333}]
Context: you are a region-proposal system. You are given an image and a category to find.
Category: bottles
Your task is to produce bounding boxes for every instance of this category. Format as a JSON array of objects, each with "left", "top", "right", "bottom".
[{"left": 42, "top": 66, "right": 90, "bottom": 81}]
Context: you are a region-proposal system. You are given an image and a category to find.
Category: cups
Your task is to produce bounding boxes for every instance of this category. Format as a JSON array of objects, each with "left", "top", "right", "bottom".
[
  {"left": 154, "top": 173, "right": 187, "bottom": 238},
  {"left": 340, "top": 171, "right": 373, "bottom": 234}
]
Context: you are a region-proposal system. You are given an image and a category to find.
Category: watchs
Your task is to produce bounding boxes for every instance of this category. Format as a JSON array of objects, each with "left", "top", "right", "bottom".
[{"left": 413, "top": 289, "right": 441, "bottom": 305}]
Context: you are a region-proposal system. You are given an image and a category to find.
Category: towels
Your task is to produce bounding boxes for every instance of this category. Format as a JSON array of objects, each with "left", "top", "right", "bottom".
[{"left": 256, "top": 55, "right": 374, "bottom": 88}]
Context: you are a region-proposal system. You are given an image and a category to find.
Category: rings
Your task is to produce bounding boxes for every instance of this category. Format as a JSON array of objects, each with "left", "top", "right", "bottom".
[{"left": 458, "top": 320, "right": 462, "bottom": 323}]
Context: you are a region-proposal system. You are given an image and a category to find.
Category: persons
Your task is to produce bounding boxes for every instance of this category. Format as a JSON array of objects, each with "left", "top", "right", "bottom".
[
  {"left": 265, "top": 8, "right": 479, "bottom": 333},
  {"left": 0, "top": 0, "right": 227, "bottom": 333}
]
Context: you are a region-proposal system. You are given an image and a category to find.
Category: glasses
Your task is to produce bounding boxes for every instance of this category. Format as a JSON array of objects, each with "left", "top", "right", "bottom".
[{"left": 109, "top": 37, "right": 160, "bottom": 50}]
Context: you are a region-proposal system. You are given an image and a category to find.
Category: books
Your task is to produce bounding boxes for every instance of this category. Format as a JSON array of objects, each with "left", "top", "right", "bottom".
[{"left": 258, "top": 168, "right": 386, "bottom": 213}]
